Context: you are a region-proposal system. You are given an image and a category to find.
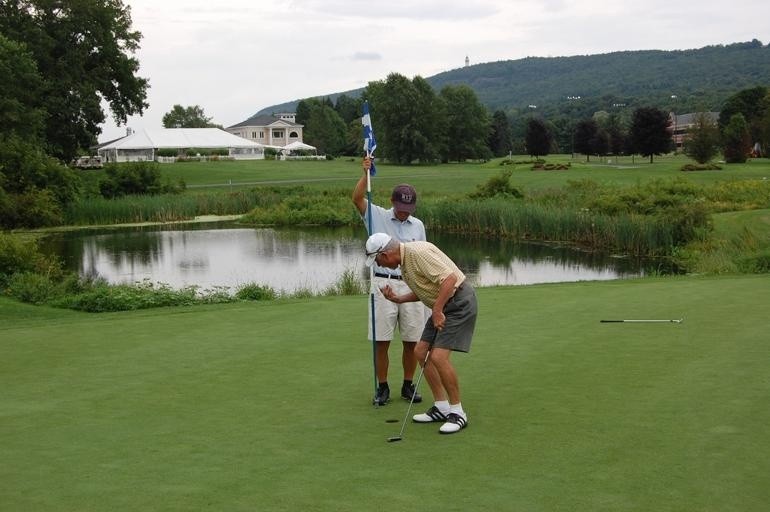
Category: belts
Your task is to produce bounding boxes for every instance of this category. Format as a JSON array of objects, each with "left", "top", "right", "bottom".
[{"left": 374, "top": 272, "right": 404, "bottom": 280}]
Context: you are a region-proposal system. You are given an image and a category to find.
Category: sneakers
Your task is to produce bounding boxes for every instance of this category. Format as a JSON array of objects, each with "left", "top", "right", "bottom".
[
  {"left": 372, "top": 387, "right": 390, "bottom": 406},
  {"left": 412, "top": 407, "right": 449, "bottom": 423},
  {"left": 400, "top": 383, "right": 423, "bottom": 403},
  {"left": 439, "top": 412, "right": 467, "bottom": 434}
]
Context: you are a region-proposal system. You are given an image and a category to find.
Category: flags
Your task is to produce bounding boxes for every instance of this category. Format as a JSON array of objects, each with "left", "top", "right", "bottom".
[{"left": 361, "top": 98, "right": 378, "bottom": 176}]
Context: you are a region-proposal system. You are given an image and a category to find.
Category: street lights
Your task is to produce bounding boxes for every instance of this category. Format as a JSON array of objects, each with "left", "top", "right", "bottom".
[
  {"left": 530, "top": 105, "right": 537, "bottom": 112},
  {"left": 611, "top": 101, "right": 626, "bottom": 115},
  {"left": 566, "top": 93, "right": 580, "bottom": 157},
  {"left": 671, "top": 93, "right": 679, "bottom": 151}
]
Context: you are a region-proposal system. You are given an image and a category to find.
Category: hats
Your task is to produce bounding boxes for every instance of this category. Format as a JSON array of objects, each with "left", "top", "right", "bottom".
[
  {"left": 391, "top": 184, "right": 417, "bottom": 213},
  {"left": 365, "top": 233, "right": 392, "bottom": 268}
]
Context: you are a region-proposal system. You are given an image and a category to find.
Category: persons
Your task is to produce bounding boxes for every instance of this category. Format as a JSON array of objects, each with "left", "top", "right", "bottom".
[
  {"left": 351, "top": 155, "right": 428, "bottom": 407},
  {"left": 364, "top": 232, "right": 479, "bottom": 436}
]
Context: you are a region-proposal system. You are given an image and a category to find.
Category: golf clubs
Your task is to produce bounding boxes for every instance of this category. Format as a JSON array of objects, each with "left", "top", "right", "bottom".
[
  {"left": 386, "top": 328, "right": 438, "bottom": 442},
  {"left": 601, "top": 318, "right": 684, "bottom": 323}
]
